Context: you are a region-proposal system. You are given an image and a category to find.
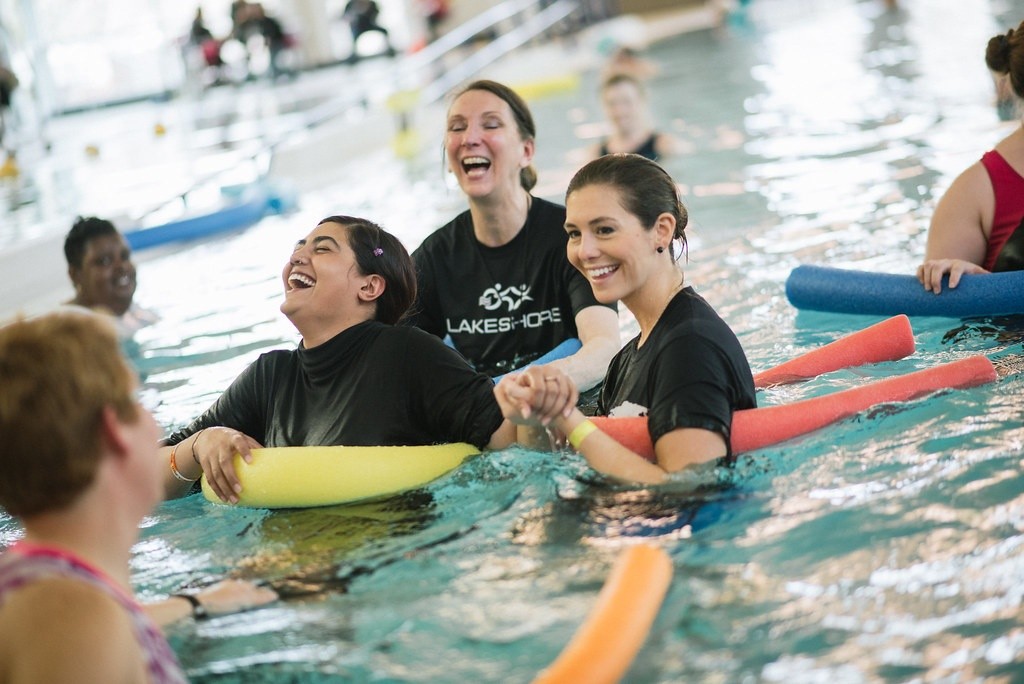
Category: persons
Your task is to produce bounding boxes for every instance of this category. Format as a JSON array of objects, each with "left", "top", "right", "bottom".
[
  {"left": 343, "top": 0, "right": 396, "bottom": 65},
  {"left": 192, "top": 0, "right": 291, "bottom": 84},
  {"left": 586, "top": 72, "right": 672, "bottom": 161},
  {"left": 64, "top": 216, "right": 160, "bottom": 331},
  {"left": 1, "top": 17, "right": 39, "bottom": 176},
  {"left": 916, "top": 22, "right": 1024, "bottom": 295},
  {"left": 0, "top": 313, "right": 278, "bottom": 684},
  {"left": 425, "top": 0, "right": 451, "bottom": 47},
  {"left": 159, "top": 216, "right": 577, "bottom": 505},
  {"left": 492, "top": 154, "right": 757, "bottom": 485},
  {"left": 404, "top": 80, "right": 620, "bottom": 406}
]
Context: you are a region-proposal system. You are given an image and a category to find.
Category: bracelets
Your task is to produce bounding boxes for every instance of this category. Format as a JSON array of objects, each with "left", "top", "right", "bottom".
[
  {"left": 171, "top": 441, "right": 201, "bottom": 482},
  {"left": 170, "top": 593, "right": 208, "bottom": 621},
  {"left": 192, "top": 429, "right": 204, "bottom": 464},
  {"left": 569, "top": 421, "right": 600, "bottom": 449}
]
